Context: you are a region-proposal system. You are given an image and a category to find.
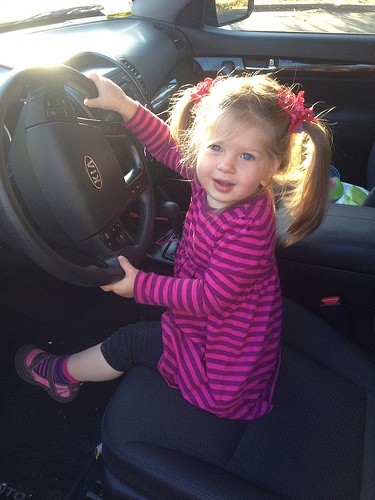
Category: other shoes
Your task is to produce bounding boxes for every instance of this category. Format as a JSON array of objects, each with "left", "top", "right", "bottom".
[{"left": 14, "top": 344, "right": 82, "bottom": 403}]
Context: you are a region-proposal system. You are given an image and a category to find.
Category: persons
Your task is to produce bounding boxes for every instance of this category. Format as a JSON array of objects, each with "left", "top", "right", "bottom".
[{"left": 13, "top": 72, "right": 333, "bottom": 421}]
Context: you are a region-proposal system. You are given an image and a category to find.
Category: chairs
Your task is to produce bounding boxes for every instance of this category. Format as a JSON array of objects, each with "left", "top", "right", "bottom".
[{"left": 91, "top": 294, "right": 375, "bottom": 500}]
